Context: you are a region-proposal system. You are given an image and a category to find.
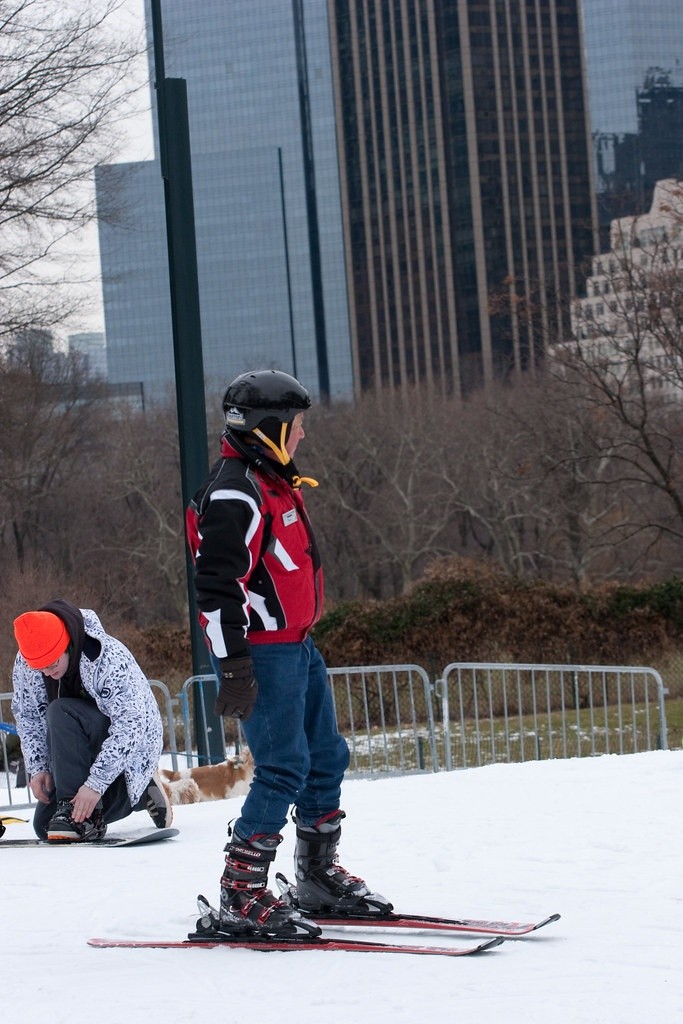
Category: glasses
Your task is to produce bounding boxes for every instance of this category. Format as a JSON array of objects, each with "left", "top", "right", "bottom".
[{"left": 45, "top": 658, "right": 59, "bottom": 668}]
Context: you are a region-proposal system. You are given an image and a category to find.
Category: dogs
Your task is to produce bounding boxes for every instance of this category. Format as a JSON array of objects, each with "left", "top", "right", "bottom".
[{"left": 158, "top": 748, "right": 254, "bottom": 805}]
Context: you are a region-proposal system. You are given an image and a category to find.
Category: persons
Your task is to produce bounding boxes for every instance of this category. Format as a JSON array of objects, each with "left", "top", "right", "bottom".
[
  {"left": 184, "top": 370, "right": 394, "bottom": 941},
  {"left": 11, "top": 598, "right": 172, "bottom": 840}
]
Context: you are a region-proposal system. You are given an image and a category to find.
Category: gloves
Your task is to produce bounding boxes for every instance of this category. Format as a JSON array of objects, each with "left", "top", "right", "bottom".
[{"left": 213, "top": 657, "right": 258, "bottom": 720}]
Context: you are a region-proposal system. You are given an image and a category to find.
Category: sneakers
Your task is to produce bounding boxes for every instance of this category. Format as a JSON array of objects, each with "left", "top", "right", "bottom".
[
  {"left": 47, "top": 800, "right": 107, "bottom": 840},
  {"left": 146, "top": 770, "right": 173, "bottom": 828}
]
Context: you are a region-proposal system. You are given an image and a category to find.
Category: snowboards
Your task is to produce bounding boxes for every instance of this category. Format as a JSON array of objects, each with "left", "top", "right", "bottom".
[{"left": 0, "top": 826, "right": 180, "bottom": 848}]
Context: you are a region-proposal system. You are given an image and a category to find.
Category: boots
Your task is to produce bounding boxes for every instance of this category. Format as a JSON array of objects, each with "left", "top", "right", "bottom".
[
  {"left": 221, "top": 832, "right": 301, "bottom": 934},
  {"left": 293, "top": 812, "right": 372, "bottom": 902}
]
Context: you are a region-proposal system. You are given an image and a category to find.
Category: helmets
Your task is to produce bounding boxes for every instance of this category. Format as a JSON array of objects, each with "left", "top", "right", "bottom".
[{"left": 223, "top": 370, "right": 311, "bottom": 430}]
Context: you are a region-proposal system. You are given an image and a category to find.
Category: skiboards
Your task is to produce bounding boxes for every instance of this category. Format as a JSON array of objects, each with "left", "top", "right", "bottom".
[{"left": 87, "top": 911, "right": 561, "bottom": 958}]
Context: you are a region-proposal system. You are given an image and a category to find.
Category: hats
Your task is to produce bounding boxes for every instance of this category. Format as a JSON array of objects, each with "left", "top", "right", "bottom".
[{"left": 13, "top": 611, "right": 70, "bottom": 669}]
